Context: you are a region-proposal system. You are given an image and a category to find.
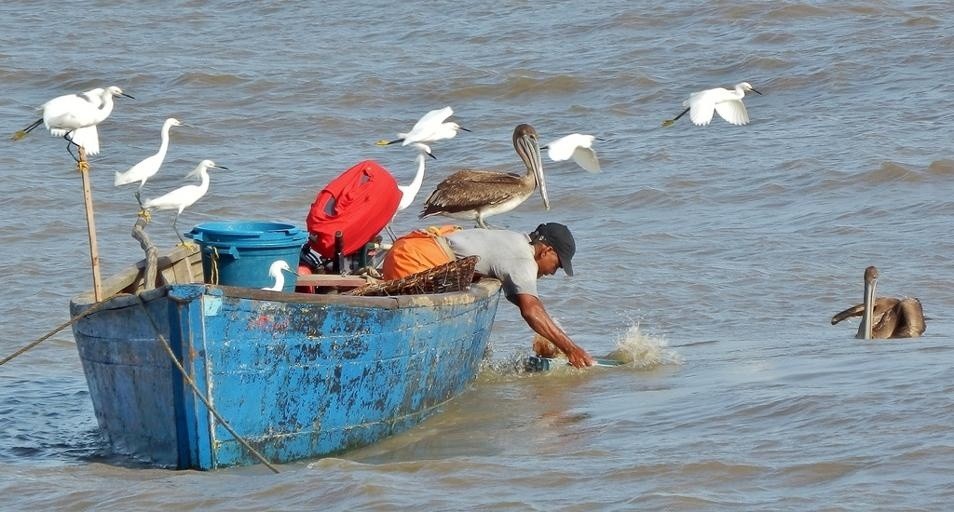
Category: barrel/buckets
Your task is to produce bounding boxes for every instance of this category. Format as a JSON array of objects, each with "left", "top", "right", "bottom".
[{"left": 185, "top": 220, "right": 309, "bottom": 292}]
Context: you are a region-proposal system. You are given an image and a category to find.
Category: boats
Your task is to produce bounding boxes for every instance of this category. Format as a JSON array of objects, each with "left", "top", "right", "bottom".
[{"left": 66, "top": 246, "right": 501, "bottom": 473}]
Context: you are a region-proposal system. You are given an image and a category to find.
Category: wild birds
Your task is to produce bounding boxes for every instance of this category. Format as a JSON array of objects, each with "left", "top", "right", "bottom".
[
  {"left": 539, "top": 133, "right": 605, "bottom": 174},
  {"left": 662, "top": 81, "right": 762, "bottom": 127},
  {"left": 259, "top": 260, "right": 301, "bottom": 292},
  {"left": 831, "top": 266, "right": 927, "bottom": 340},
  {"left": 7, "top": 85, "right": 234, "bottom": 252},
  {"left": 366, "top": 102, "right": 473, "bottom": 243},
  {"left": 417, "top": 124, "right": 550, "bottom": 229}
]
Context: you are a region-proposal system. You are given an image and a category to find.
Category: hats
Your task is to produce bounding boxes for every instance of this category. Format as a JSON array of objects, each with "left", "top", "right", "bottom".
[{"left": 537, "top": 221, "right": 578, "bottom": 277}]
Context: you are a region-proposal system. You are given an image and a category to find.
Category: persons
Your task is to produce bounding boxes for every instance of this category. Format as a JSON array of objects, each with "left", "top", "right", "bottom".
[{"left": 381, "top": 220, "right": 596, "bottom": 367}]
